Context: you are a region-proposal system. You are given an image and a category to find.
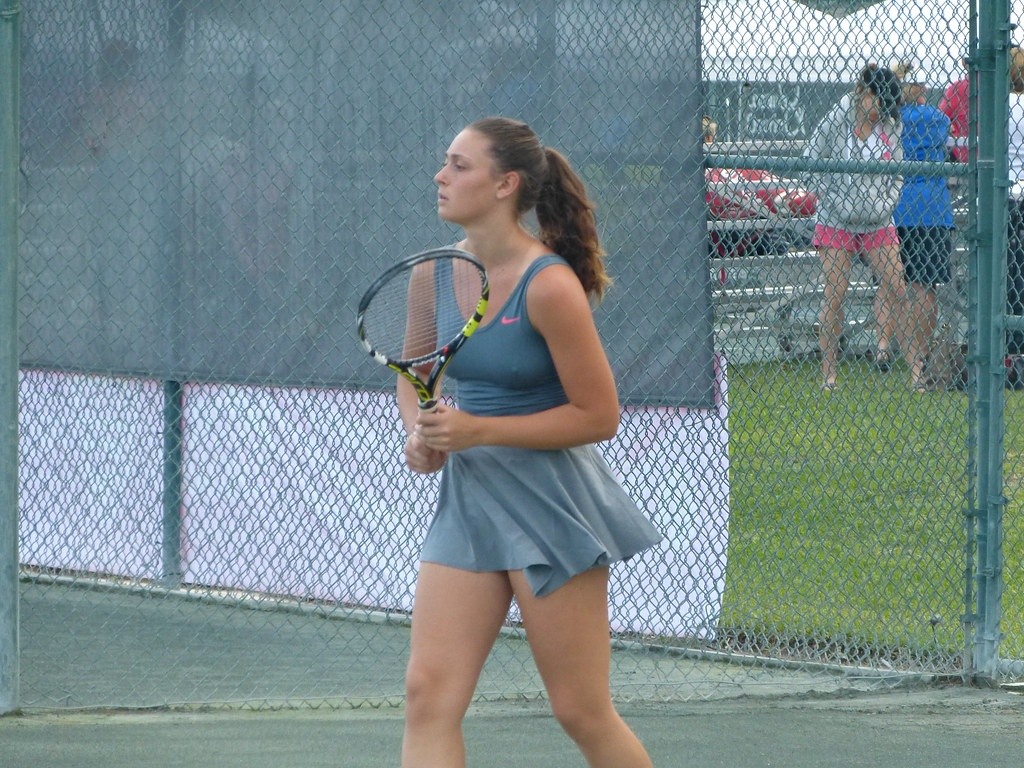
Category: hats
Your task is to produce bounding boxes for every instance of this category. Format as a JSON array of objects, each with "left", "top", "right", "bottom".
[{"left": 869, "top": 69, "right": 901, "bottom": 118}]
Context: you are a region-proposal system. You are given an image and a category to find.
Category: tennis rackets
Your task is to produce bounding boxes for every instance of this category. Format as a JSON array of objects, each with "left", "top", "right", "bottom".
[{"left": 356, "top": 248, "right": 490, "bottom": 428}]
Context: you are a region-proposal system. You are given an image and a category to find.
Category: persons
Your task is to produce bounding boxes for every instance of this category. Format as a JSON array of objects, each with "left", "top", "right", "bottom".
[
  {"left": 395, "top": 117, "right": 665, "bottom": 768},
  {"left": 803, "top": 48, "right": 1024, "bottom": 394},
  {"left": 701, "top": 119, "right": 717, "bottom": 142}
]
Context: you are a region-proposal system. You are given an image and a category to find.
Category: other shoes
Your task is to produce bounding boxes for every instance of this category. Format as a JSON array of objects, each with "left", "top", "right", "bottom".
[
  {"left": 824, "top": 376, "right": 839, "bottom": 391},
  {"left": 911, "top": 379, "right": 927, "bottom": 395},
  {"left": 874, "top": 349, "right": 889, "bottom": 371}
]
